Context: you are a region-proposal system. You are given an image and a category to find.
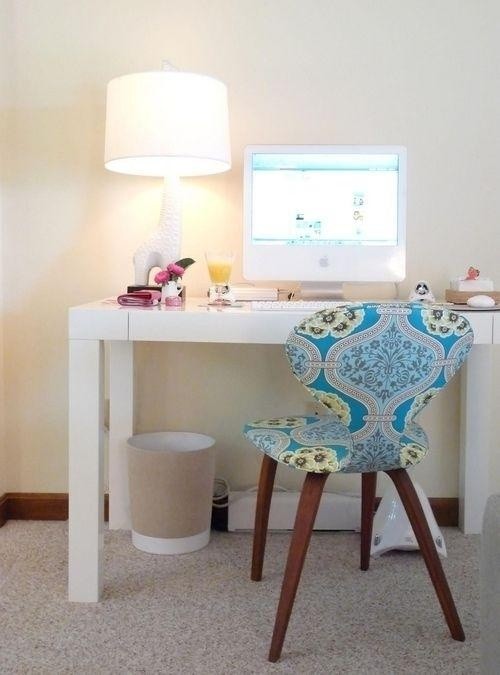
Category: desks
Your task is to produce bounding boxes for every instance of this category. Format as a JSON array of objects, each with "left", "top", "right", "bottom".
[{"left": 64, "top": 296, "right": 500, "bottom": 604}]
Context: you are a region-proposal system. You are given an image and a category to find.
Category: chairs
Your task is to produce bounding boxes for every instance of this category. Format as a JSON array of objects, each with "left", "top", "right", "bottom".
[{"left": 243, "top": 303, "right": 475, "bottom": 665}]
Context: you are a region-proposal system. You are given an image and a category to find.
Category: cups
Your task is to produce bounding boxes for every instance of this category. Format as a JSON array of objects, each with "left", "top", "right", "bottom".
[
  {"left": 206, "top": 251, "right": 236, "bottom": 284},
  {"left": 161, "top": 280, "right": 183, "bottom": 303}
]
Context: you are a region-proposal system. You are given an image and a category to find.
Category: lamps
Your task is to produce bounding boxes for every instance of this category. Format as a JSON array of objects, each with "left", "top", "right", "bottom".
[{"left": 101, "top": 67, "right": 236, "bottom": 299}]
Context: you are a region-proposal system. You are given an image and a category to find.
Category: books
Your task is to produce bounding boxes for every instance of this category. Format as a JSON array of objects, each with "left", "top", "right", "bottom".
[{"left": 231, "top": 289, "right": 280, "bottom": 302}]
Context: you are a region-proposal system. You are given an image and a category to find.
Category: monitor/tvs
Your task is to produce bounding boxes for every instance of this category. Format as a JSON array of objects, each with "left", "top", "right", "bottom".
[{"left": 243, "top": 144, "right": 408, "bottom": 299}]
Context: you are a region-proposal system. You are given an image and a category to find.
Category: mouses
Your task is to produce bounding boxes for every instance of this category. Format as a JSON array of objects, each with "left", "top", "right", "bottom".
[{"left": 467, "top": 294, "right": 495, "bottom": 308}]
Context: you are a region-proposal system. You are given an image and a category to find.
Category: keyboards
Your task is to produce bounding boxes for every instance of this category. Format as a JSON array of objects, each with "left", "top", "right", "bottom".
[{"left": 250, "top": 300, "right": 360, "bottom": 310}]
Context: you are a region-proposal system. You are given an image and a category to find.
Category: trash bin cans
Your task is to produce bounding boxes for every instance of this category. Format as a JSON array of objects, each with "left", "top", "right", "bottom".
[{"left": 126, "top": 431, "right": 216, "bottom": 555}]
